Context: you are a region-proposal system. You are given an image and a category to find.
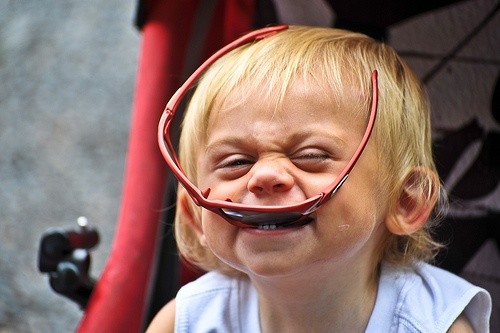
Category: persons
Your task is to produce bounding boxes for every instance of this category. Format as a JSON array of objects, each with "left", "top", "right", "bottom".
[{"left": 144, "top": 25, "right": 492, "bottom": 333}]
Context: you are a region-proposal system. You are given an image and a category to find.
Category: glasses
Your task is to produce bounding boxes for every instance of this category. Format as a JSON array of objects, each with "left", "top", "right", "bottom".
[{"left": 157, "top": 25, "right": 378, "bottom": 227}]
